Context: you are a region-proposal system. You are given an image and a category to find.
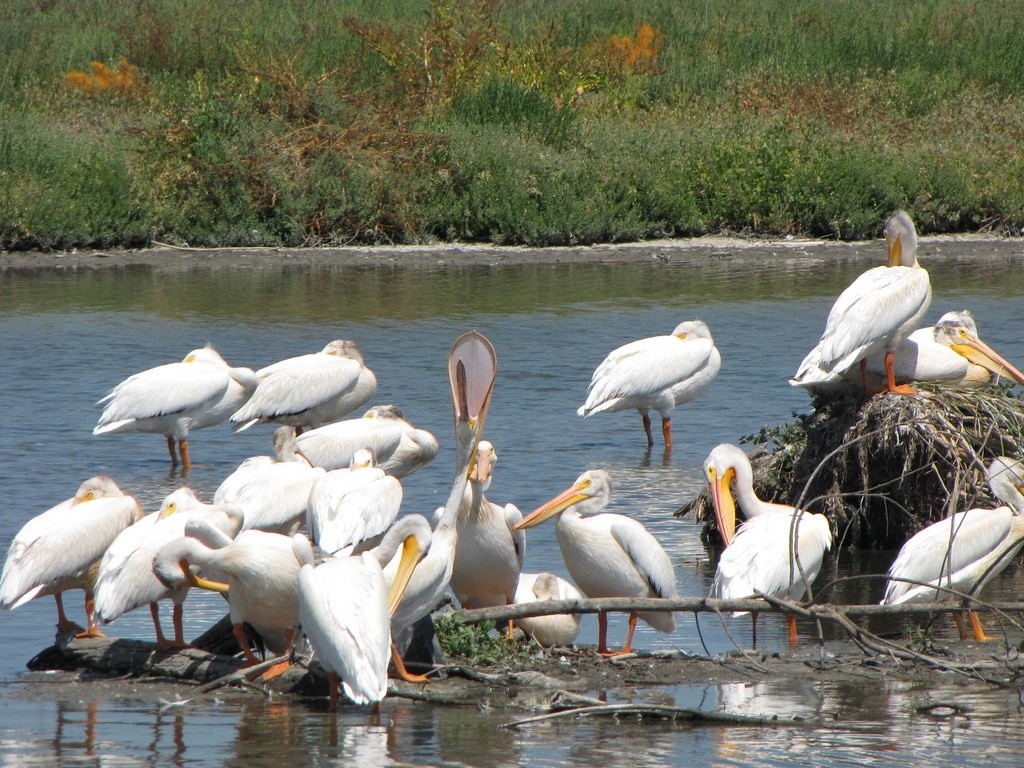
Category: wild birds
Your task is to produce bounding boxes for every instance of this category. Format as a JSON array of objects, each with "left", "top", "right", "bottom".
[
  {"left": 787, "top": 308, "right": 1024, "bottom": 402},
  {"left": 700, "top": 442, "right": 833, "bottom": 648},
  {"left": 817, "top": 207, "right": 935, "bottom": 397},
  {"left": 1, "top": 329, "right": 682, "bottom": 716},
  {"left": 576, "top": 319, "right": 723, "bottom": 448},
  {"left": 880, "top": 455, "right": 1024, "bottom": 642}
]
